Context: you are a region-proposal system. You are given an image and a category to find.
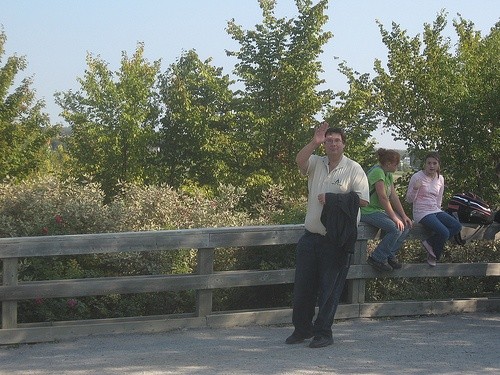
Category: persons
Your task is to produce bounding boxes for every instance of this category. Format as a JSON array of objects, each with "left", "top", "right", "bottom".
[
  {"left": 359, "top": 148, "right": 413, "bottom": 273},
  {"left": 404, "top": 156, "right": 462, "bottom": 266},
  {"left": 286, "top": 123, "right": 370, "bottom": 348}
]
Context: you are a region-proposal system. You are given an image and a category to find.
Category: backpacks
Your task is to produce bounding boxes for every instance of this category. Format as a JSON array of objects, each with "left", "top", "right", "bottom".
[{"left": 447, "top": 192, "right": 494, "bottom": 246}]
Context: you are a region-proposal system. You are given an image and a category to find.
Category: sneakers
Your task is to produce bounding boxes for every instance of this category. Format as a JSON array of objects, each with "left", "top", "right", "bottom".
[
  {"left": 387, "top": 259, "right": 404, "bottom": 269},
  {"left": 309, "top": 333, "right": 333, "bottom": 348},
  {"left": 422, "top": 240, "right": 437, "bottom": 259},
  {"left": 367, "top": 256, "right": 392, "bottom": 273},
  {"left": 425, "top": 253, "right": 437, "bottom": 266},
  {"left": 286, "top": 325, "right": 315, "bottom": 344}
]
